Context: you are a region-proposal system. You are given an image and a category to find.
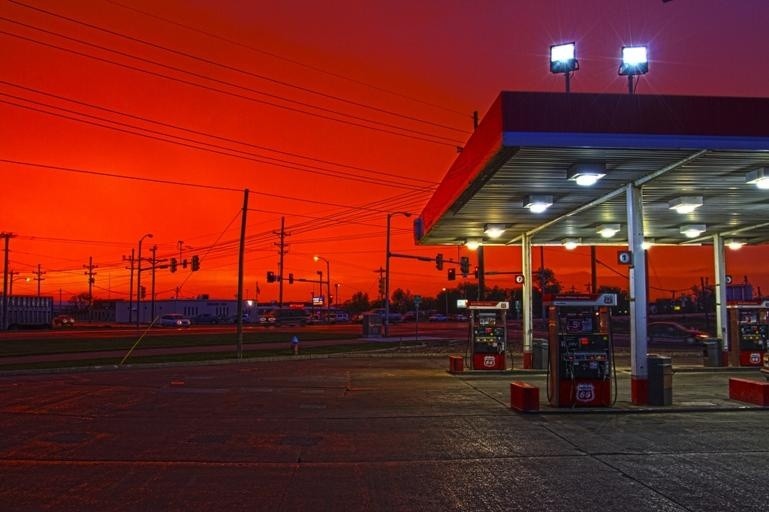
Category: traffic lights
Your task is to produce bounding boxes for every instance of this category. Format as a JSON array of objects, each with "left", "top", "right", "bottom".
[
  {"left": 460, "top": 255, "right": 469, "bottom": 274},
  {"left": 266, "top": 271, "right": 275, "bottom": 283},
  {"left": 289, "top": 272, "right": 294, "bottom": 285},
  {"left": 140, "top": 286, "right": 146, "bottom": 298},
  {"left": 170, "top": 257, "right": 178, "bottom": 273},
  {"left": 191, "top": 255, "right": 200, "bottom": 272},
  {"left": 448, "top": 267, "right": 456, "bottom": 280},
  {"left": 435, "top": 253, "right": 443, "bottom": 271}
]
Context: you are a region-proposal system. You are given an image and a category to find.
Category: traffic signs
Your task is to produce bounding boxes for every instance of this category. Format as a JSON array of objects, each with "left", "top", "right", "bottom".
[{"left": 475, "top": 266, "right": 479, "bottom": 279}]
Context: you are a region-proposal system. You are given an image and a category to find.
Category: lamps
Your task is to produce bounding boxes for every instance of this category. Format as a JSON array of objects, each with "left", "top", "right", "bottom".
[
  {"left": 680, "top": 225, "right": 707, "bottom": 238},
  {"left": 724, "top": 238, "right": 748, "bottom": 251},
  {"left": 669, "top": 196, "right": 704, "bottom": 214},
  {"left": 567, "top": 164, "right": 606, "bottom": 185},
  {"left": 523, "top": 195, "right": 554, "bottom": 214},
  {"left": 562, "top": 238, "right": 583, "bottom": 251},
  {"left": 550, "top": 42, "right": 649, "bottom": 77},
  {"left": 463, "top": 238, "right": 483, "bottom": 252},
  {"left": 484, "top": 224, "right": 505, "bottom": 238},
  {"left": 644, "top": 238, "right": 656, "bottom": 247},
  {"left": 745, "top": 168, "right": 769, "bottom": 190},
  {"left": 595, "top": 223, "right": 621, "bottom": 238}
]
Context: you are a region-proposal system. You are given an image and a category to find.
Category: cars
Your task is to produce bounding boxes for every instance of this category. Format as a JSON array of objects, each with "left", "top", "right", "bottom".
[
  {"left": 613, "top": 305, "right": 628, "bottom": 316},
  {"left": 306, "top": 308, "right": 471, "bottom": 324},
  {"left": 51, "top": 314, "right": 80, "bottom": 330},
  {"left": 190, "top": 313, "right": 277, "bottom": 325},
  {"left": 647, "top": 321, "right": 712, "bottom": 347},
  {"left": 158, "top": 314, "right": 191, "bottom": 329}
]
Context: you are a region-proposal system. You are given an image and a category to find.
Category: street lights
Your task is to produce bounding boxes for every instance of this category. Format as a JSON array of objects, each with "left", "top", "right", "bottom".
[
  {"left": 550, "top": 41, "right": 577, "bottom": 95},
  {"left": 442, "top": 288, "right": 449, "bottom": 315},
  {"left": 313, "top": 256, "right": 331, "bottom": 322},
  {"left": 383, "top": 211, "right": 412, "bottom": 335},
  {"left": 10, "top": 272, "right": 31, "bottom": 325},
  {"left": 334, "top": 283, "right": 340, "bottom": 308},
  {"left": 136, "top": 233, "right": 154, "bottom": 328},
  {"left": 619, "top": 43, "right": 650, "bottom": 95}
]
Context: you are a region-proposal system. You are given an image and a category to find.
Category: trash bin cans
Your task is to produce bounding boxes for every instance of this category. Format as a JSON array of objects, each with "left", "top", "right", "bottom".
[
  {"left": 533, "top": 339, "right": 549, "bottom": 369},
  {"left": 363, "top": 313, "right": 384, "bottom": 337},
  {"left": 647, "top": 353, "right": 673, "bottom": 406},
  {"left": 702, "top": 339, "right": 723, "bottom": 366}
]
